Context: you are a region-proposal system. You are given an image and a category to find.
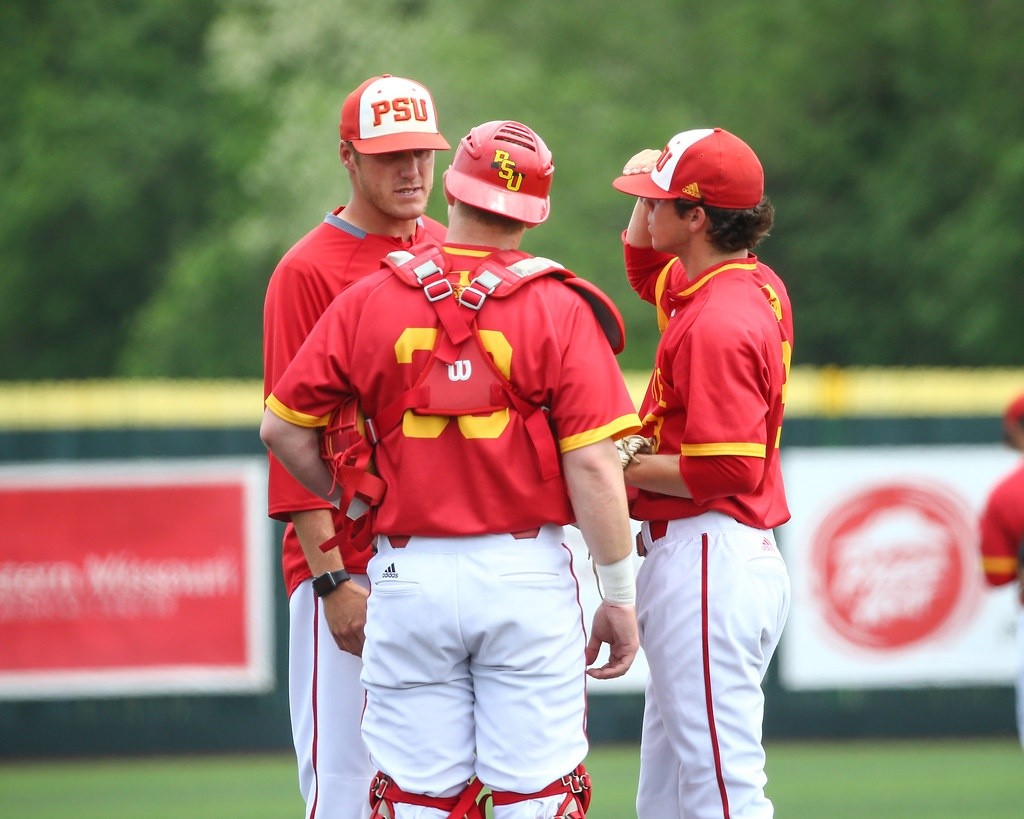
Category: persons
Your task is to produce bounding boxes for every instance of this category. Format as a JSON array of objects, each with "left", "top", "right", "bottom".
[
  {"left": 979, "top": 395, "right": 1024, "bottom": 758},
  {"left": 260, "top": 119, "right": 646, "bottom": 818},
  {"left": 262, "top": 74, "right": 447, "bottom": 818},
  {"left": 618, "top": 124, "right": 793, "bottom": 817}
]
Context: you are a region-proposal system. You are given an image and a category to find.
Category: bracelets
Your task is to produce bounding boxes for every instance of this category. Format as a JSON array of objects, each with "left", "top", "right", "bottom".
[
  {"left": 328, "top": 492, "right": 369, "bottom": 522},
  {"left": 594, "top": 550, "right": 637, "bottom": 603}
]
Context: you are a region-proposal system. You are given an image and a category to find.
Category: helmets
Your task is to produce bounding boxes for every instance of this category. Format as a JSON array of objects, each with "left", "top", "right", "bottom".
[{"left": 445, "top": 120, "right": 554, "bottom": 226}]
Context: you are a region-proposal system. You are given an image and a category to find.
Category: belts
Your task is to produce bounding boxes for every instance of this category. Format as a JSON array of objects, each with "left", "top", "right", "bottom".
[
  {"left": 388, "top": 529, "right": 538, "bottom": 548},
  {"left": 635, "top": 520, "right": 669, "bottom": 558}
]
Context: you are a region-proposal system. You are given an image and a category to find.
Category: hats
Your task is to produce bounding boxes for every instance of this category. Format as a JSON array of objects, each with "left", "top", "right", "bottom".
[
  {"left": 340, "top": 73, "right": 452, "bottom": 154},
  {"left": 613, "top": 127, "right": 766, "bottom": 209}
]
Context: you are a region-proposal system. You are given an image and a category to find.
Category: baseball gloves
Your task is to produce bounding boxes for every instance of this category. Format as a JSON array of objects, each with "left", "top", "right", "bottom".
[{"left": 612, "top": 434, "right": 656, "bottom": 470}]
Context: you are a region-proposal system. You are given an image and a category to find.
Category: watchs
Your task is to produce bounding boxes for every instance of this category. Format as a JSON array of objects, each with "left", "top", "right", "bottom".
[{"left": 313, "top": 568, "right": 350, "bottom": 597}]
